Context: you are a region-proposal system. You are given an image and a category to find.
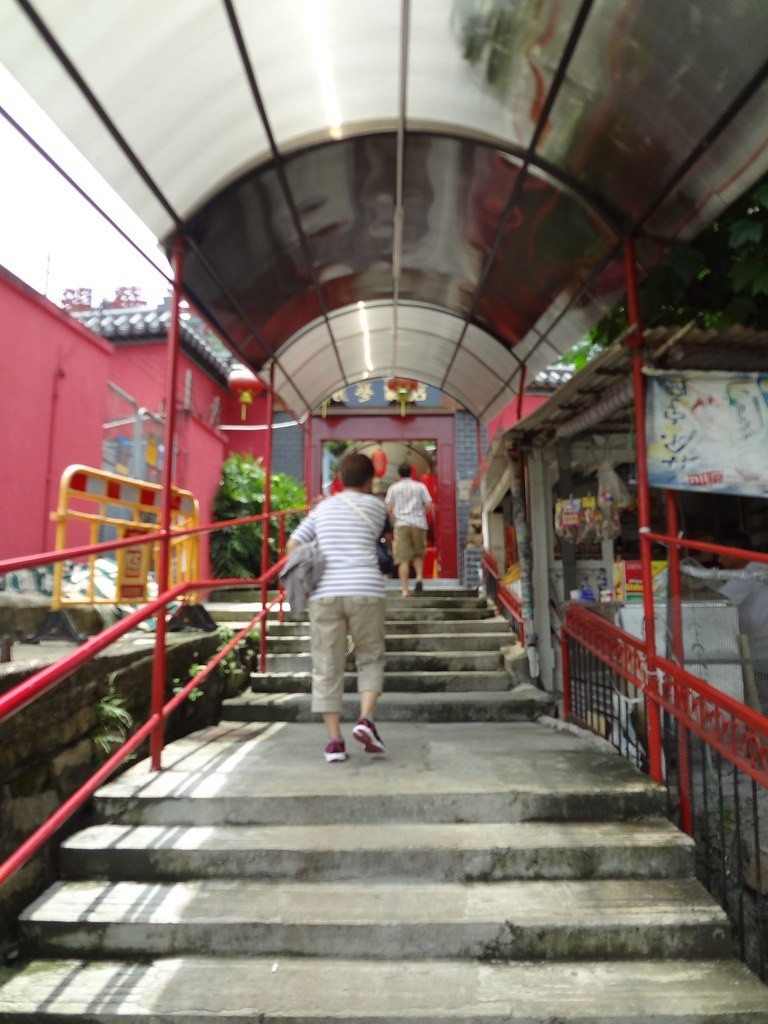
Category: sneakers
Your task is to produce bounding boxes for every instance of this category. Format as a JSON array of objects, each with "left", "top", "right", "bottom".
[
  {"left": 352, "top": 718, "right": 386, "bottom": 754},
  {"left": 323, "top": 739, "right": 347, "bottom": 763}
]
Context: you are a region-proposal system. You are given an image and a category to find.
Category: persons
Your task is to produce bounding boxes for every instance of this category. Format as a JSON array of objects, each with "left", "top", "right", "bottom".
[
  {"left": 285, "top": 453, "right": 391, "bottom": 764},
  {"left": 384, "top": 462, "right": 433, "bottom": 598}
]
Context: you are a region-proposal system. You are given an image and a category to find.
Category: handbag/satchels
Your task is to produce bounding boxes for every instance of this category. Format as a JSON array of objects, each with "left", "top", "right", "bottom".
[{"left": 376, "top": 534, "right": 395, "bottom": 574}]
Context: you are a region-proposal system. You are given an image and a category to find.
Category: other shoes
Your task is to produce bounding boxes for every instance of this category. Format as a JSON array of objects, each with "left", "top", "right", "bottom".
[{"left": 415, "top": 581, "right": 422, "bottom": 592}]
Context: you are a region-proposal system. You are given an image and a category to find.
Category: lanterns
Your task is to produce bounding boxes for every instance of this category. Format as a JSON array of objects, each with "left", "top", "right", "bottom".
[
  {"left": 388, "top": 375, "right": 418, "bottom": 398},
  {"left": 372, "top": 451, "right": 387, "bottom": 478},
  {"left": 329, "top": 478, "right": 344, "bottom": 497},
  {"left": 228, "top": 369, "right": 266, "bottom": 403},
  {"left": 409, "top": 464, "right": 435, "bottom": 527}
]
